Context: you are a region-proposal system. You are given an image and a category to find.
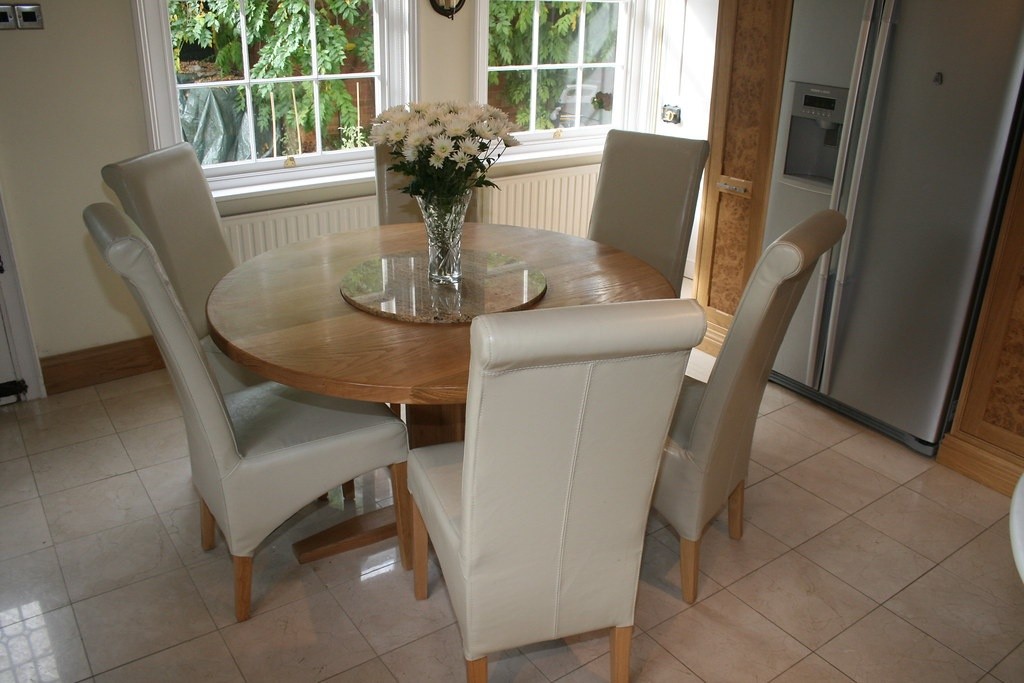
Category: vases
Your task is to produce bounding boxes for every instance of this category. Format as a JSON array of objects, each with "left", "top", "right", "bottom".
[{"left": 414, "top": 186, "right": 475, "bottom": 284}]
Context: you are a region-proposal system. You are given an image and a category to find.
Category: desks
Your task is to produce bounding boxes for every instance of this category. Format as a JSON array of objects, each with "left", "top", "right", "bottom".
[{"left": 205, "top": 221, "right": 677, "bottom": 566}]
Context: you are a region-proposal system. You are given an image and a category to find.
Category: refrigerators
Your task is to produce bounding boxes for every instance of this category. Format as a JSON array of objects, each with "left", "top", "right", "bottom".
[{"left": 764, "top": 0, "right": 1024, "bottom": 461}]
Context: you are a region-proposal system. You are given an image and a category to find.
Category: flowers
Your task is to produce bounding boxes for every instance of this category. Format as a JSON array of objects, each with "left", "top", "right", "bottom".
[{"left": 368, "top": 98, "right": 521, "bottom": 194}]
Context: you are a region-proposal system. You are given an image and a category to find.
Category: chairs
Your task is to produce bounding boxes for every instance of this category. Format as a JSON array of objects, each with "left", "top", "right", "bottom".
[{"left": 82, "top": 129, "right": 849, "bottom": 682}]
